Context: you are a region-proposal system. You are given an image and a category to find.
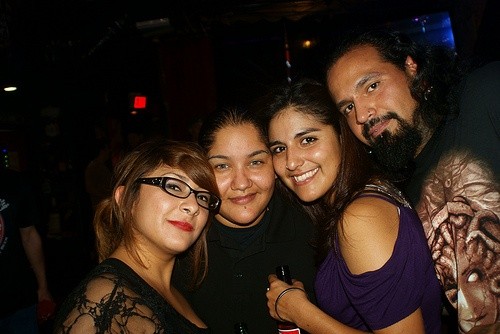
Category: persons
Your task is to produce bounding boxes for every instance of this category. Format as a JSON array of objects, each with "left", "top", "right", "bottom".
[
  {"left": 326, "top": 31, "right": 500, "bottom": 334},
  {"left": 170, "top": 105, "right": 317, "bottom": 334},
  {"left": 48, "top": 139, "right": 222, "bottom": 334},
  {"left": 265, "top": 81, "right": 443, "bottom": 334},
  {"left": 0, "top": 178, "right": 57, "bottom": 334}
]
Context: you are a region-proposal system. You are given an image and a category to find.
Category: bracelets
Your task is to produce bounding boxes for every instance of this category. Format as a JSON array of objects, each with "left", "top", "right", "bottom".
[
  {"left": 274, "top": 287, "right": 310, "bottom": 323},
  {"left": 274, "top": 287, "right": 311, "bottom": 323}
]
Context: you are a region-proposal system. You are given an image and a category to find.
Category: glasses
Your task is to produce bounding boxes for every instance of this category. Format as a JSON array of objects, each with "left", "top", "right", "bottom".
[{"left": 136, "top": 177, "right": 222, "bottom": 212}]
